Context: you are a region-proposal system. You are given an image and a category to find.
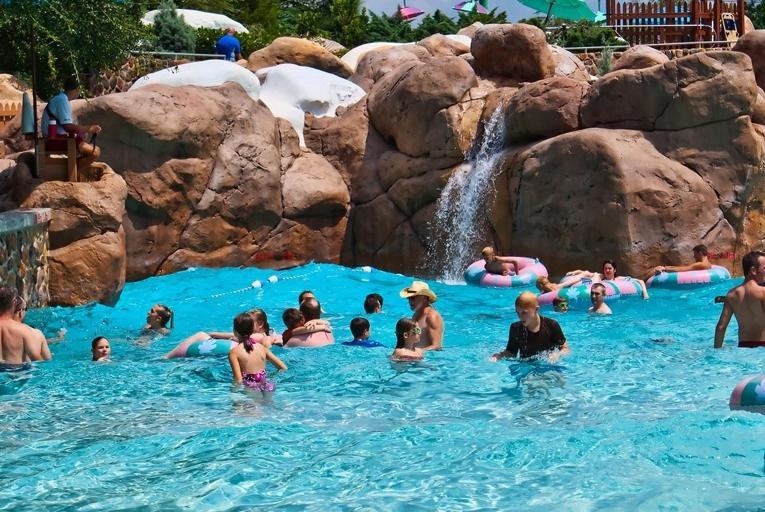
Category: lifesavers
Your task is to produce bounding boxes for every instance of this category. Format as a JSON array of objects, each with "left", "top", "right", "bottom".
[
  {"left": 645, "top": 264, "right": 731, "bottom": 289},
  {"left": 464, "top": 256, "right": 548, "bottom": 287},
  {"left": 538, "top": 275, "right": 643, "bottom": 305},
  {"left": 730, "top": 376, "right": 765, "bottom": 405},
  {"left": 174, "top": 339, "right": 238, "bottom": 356}
]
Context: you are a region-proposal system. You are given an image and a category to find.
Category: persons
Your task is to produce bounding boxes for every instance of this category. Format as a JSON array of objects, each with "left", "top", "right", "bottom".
[
  {"left": 661, "top": 244, "right": 712, "bottom": 271},
  {"left": 566, "top": 258, "right": 651, "bottom": 302},
  {"left": 553, "top": 296, "right": 568, "bottom": 313},
  {"left": 585, "top": 283, "right": 613, "bottom": 314},
  {"left": 481, "top": 246, "right": 519, "bottom": 277},
  {"left": 0, "top": 283, "right": 42, "bottom": 365},
  {"left": 536, "top": 270, "right": 603, "bottom": 293},
  {"left": 638, "top": 264, "right": 664, "bottom": 281},
  {"left": 90, "top": 336, "right": 114, "bottom": 363},
  {"left": 127, "top": 305, "right": 174, "bottom": 346},
  {"left": 40, "top": 77, "right": 102, "bottom": 168},
  {"left": 215, "top": 26, "right": 244, "bottom": 61},
  {"left": 12, "top": 294, "right": 52, "bottom": 361},
  {"left": 163, "top": 281, "right": 446, "bottom": 398},
  {"left": 714, "top": 251, "right": 765, "bottom": 348},
  {"left": 488, "top": 290, "right": 569, "bottom": 402}
]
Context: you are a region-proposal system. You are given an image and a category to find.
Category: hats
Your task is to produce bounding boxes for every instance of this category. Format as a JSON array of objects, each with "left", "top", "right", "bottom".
[{"left": 400, "top": 281, "right": 437, "bottom": 303}]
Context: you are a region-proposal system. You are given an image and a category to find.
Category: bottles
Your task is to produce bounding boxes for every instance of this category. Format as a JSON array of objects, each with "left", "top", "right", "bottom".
[
  {"left": 230, "top": 51, "right": 236, "bottom": 61},
  {"left": 48, "top": 119, "right": 57, "bottom": 138}
]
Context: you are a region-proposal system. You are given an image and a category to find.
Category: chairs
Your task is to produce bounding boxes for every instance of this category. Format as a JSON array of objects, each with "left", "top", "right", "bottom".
[{"left": 21, "top": 91, "right": 78, "bottom": 181}]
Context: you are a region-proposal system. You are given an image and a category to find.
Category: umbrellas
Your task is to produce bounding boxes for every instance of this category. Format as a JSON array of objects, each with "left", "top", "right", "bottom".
[
  {"left": 452, "top": 1, "right": 490, "bottom": 14},
  {"left": 518, "top": 0, "right": 599, "bottom": 25},
  {"left": 388, "top": 5, "right": 429, "bottom": 23}
]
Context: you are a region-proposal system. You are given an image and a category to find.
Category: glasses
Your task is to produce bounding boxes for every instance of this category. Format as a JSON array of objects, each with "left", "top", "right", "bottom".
[
  {"left": 558, "top": 303, "right": 568, "bottom": 307},
  {"left": 406, "top": 328, "right": 421, "bottom": 335}
]
